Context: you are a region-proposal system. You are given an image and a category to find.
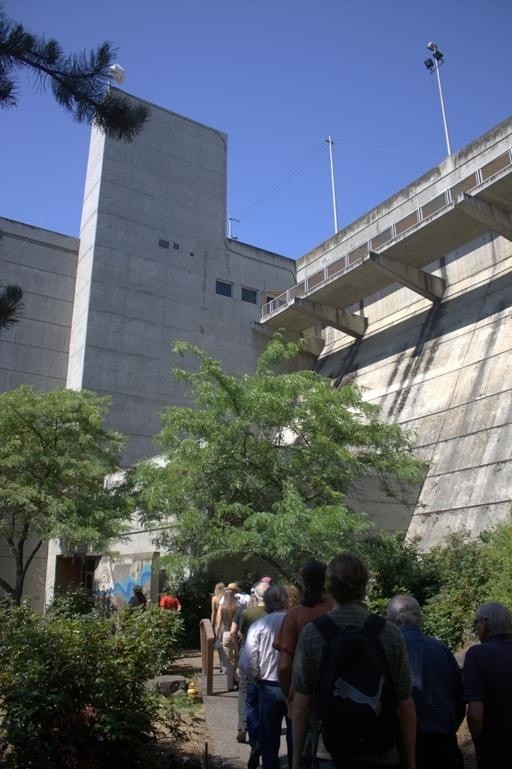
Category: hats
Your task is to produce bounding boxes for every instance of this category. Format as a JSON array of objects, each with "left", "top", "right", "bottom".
[
  {"left": 262, "top": 576, "right": 273, "bottom": 584},
  {"left": 224, "top": 583, "right": 241, "bottom": 591}
]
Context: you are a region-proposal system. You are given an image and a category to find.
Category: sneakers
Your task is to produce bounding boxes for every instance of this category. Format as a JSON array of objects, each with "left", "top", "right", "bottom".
[
  {"left": 248, "top": 744, "right": 262, "bottom": 768},
  {"left": 237, "top": 728, "right": 247, "bottom": 742},
  {"left": 229, "top": 681, "right": 238, "bottom": 692}
]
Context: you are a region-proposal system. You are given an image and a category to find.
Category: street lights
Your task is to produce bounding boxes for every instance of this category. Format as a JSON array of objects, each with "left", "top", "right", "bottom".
[{"left": 424, "top": 42, "right": 451, "bottom": 158}]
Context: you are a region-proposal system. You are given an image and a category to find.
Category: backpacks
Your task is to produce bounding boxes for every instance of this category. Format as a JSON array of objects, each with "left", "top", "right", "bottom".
[{"left": 311, "top": 614, "right": 397, "bottom": 760}]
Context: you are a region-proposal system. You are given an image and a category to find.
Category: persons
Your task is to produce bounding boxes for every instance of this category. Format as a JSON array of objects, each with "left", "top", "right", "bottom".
[
  {"left": 128, "top": 585, "right": 146, "bottom": 616},
  {"left": 211, "top": 552, "right": 335, "bottom": 769},
  {"left": 291, "top": 552, "right": 417, "bottom": 769},
  {"left": 464, "top": 603, "right": 512, "bottom": 769},
  {"left": 387, "top": 594, "right": 466, "bottom": 769},
  {"left": 160, "top": 588, "right": 181, "bottom": 612}
]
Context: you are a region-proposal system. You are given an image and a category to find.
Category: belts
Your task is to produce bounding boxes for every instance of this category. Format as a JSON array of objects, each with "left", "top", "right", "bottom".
[{"left": 263, "top": 680, "right": 279, "bottom": 687}]
